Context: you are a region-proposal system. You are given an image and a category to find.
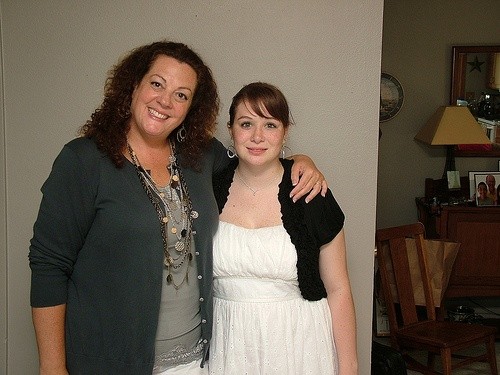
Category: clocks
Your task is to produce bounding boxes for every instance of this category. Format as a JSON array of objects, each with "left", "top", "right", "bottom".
[{"left": 380, "top": 71, "right": 405, "bottom": 123}]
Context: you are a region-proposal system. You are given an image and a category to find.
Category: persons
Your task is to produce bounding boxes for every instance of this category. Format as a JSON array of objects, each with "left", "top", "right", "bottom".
[
  {"left": 477, "top": 182, "right": 493, "bottom": 206},
  {"left": 27, "top": 41, "right": 326, "bottom": 375},
  {"left": 497, "top": 184, "right": 500, "bottom": 206},
  {"left": 486, "top": 175, "right": 498, "bottom": 205},
  {"left": 208, "top": 80, "right": 360, "bottom": 375}
]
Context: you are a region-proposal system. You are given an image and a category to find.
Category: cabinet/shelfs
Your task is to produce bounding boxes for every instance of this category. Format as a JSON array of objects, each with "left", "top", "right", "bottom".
[{"left": 415, "top": 197, "right": 500, "bottom": 298}]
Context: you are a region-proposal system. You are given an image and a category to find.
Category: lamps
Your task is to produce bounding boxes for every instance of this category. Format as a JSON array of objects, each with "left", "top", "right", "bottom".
[{"left": 415, "top": 105, "right": 492, "bottom": 192}]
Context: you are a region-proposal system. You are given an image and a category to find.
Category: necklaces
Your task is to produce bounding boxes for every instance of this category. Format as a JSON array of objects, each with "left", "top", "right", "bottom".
[
  {"left": 125, "top": 140, "right": 201, "bottom": 291},
  {"left": 235, "top": 162, "right": 284, "bottom": 196}
]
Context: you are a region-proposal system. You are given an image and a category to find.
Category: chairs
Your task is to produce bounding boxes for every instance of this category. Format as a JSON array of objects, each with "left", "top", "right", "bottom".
[{"left": 373, "top": 222, "right": 498, "bottom": 375}]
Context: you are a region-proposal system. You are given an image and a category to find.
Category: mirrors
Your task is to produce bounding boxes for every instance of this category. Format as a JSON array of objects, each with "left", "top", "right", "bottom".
[{"left": 450, "top": 45, "right": 500, "bottom": 157}]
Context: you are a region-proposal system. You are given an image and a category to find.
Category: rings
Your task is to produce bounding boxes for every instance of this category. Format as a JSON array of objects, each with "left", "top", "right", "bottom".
[{"left": 314, "top": 182, "right": 322, "bottom": 185}]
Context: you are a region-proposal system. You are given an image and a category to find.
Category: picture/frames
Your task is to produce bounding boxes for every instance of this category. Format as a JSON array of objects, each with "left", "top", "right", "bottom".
[
  {"left": 468, "top": 171, "right": 500, "bottom": 200},
  {"left": 474, "top": 173, "right": 500, "bottom": 208}
]
